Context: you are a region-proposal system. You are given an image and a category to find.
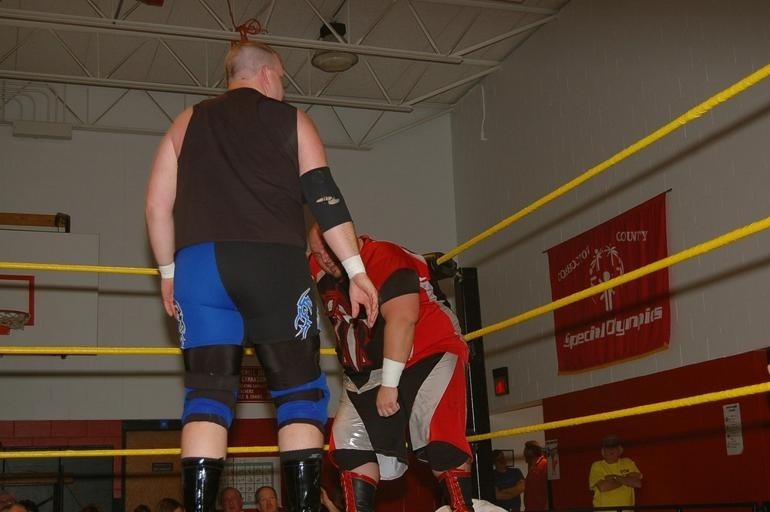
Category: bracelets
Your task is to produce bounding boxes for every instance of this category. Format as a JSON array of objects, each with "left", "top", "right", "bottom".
[
  {"left": 338, "top": 251, "right": 371, "bottom": 279},
  {"left": 154, "top": 262, "right": 178, "bottom": 281},
  {"left": 379, "top": 356, "right": 408, "bottom": 389}
]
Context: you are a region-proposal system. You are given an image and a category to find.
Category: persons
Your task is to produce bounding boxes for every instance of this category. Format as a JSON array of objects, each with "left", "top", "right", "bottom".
[
  {"left": 308, "top": 217, "right": 473, "bottom": 511},
  {"left": 485, "top": 448, "right": 527, "bottom": 512},
  {"left": 589, "top": 434, "right": 644, "bottom": 512},
  {"left": 219, "top": 486, "right": 244, "bottom": 512},
  {"left": 1, "top": 498, "right": 35, "bottom": 512},
  {"left": 255, "top": 485, "right": 279, "bottom": 512},
  {"left": 155, "top": 497, "right": 185, "bottom": 512},
  {"left": 522, "top": 440, "right": 549, "bottom": 512},
  {"left": 141, "top": 36, "right": 379, "bottom": 511},
  {"left": 320, "top": 487, "right": 346, "bottom": 511}
]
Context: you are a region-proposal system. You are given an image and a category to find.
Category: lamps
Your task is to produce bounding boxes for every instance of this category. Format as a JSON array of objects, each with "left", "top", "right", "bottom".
[{"left": 311, "top": 22, "right": 358, "bottom": 74}]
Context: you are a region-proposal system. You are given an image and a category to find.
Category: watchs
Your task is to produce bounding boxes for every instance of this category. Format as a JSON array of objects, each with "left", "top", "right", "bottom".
[{"left": 613, "top": 474, "right": 618, "bottom": 480}]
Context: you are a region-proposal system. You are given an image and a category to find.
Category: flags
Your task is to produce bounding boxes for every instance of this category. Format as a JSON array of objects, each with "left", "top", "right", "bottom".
[{"left": 546, "top": 191, "right": 672, "bottom": 377}]
